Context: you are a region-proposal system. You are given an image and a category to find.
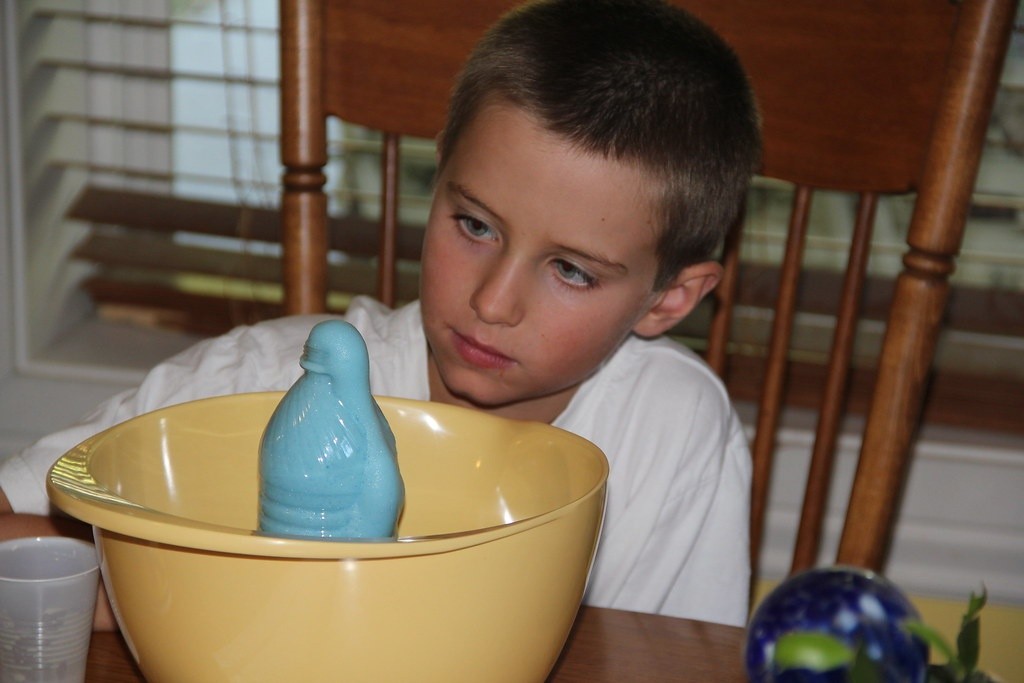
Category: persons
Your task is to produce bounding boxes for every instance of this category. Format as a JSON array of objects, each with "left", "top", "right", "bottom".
[{"left": 2, "top": 0, "right": 754, "bottom": 629}]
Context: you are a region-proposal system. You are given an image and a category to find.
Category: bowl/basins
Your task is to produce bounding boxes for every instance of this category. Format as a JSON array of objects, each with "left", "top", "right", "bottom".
[{"left": 45, "top": 391, "right": 612, "bottom": 683}]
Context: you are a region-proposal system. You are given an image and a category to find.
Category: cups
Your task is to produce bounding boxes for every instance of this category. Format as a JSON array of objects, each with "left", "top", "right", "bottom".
[{"left": 0, "top": 535, "right": 99, "bottom": 683}]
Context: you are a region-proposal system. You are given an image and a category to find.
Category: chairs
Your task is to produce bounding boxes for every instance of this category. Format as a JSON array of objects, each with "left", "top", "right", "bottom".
[{"left": 277, "top": 0, "right": 1016, "bottom": 622}]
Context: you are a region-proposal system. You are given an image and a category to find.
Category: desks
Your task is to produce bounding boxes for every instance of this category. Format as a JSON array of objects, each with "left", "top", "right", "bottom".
[{"left": 91, "top": 603, "right": 757, "bottom": 682}]
